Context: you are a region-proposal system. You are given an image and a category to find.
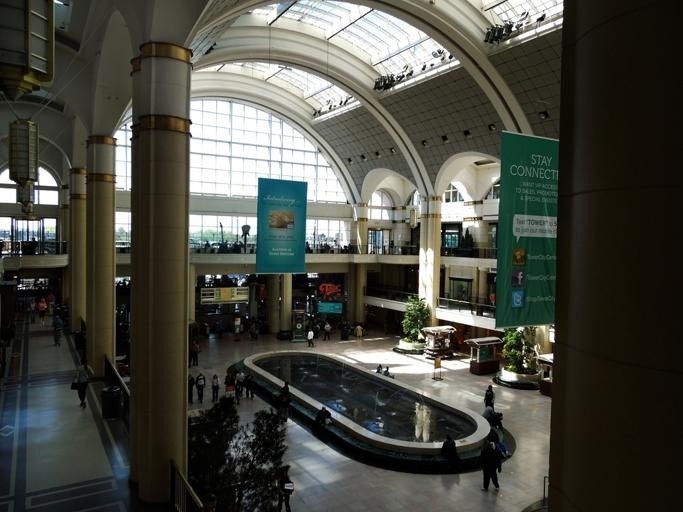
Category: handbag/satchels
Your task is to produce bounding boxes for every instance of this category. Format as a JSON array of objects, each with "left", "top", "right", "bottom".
[{"left": 71, "top": 383, "right": 80, "bottom": 389}]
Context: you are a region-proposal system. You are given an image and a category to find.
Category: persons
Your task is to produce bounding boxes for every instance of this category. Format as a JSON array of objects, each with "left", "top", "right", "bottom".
[
  {"left": 191, "top": 339, "right": 198, "bottom": 366},
  {"left": 482, "top": 383, "right": 494, "bottom": 410},
  {"left": 375, "top": 363, "right": 381, "bottom": 373},
  {"left": 305, "top": 318, "right": 363, "bottom": 341},
  {"left": 440, "top": 435, "right": 455, "bottom": 456},
  {"left": 247, "top": 316, "right": 269, "bottom": 344},
  {"left": 485, "top": 411, "right": 503, "bottom": 430},
  {"left": 50, "top": 315, "right": 62, "bottom": 347},
  {"left": 312, "top": 407, "right": 331, "bottom": 435},
  {"left": 307, "top": 328, "right": 313, "bottom": 347},
  {"left": 187, "top": 366, "right": 255, "bottom": 405},
  {"left": 383, "top": 365, "right": 394, "bottom": 378},
  {"left": 475, "top": 439, "right": 502, "bottom": 492},
  {"left": 75, "top": 357, "right": 95, "bottom": 409},
  {"left": 487, "top": 428, "right": 511, "bottom": 458},
  {"left": 482, "top": 403, "right": 494, "bottom": 419},
  {"left": 27, "top": 287, "right": 55, "bottom": 329},
  {"left": 276, "top": 380, "right": 288, "bottom": 422}
]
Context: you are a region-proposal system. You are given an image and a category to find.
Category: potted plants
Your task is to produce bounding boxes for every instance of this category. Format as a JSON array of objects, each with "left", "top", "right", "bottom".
[
  {"left": 396, "top": 295, "right": 432, "bottom": 351},
  {"left": 498, "top": 326, "right": 545, "bottom": 386}
]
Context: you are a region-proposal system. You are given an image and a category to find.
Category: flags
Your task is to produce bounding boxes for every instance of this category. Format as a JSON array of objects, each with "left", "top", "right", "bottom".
[
  {"left": 493, "top": 130, "right": 560, "bottom": 329},
  {"left": 255, "top": 177, "right": 307, "bottom": 275}
]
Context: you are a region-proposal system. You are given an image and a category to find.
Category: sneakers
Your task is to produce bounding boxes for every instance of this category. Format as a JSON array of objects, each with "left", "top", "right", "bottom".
[
  {"left": 481, "top": 488, "right": 487, "bottom": 491},
  {"left": 494, "top": 488, "right": 499, "bottom": 492},
  {"left": 80, "top": 401, "right": 86, "bottom": 408}
]
